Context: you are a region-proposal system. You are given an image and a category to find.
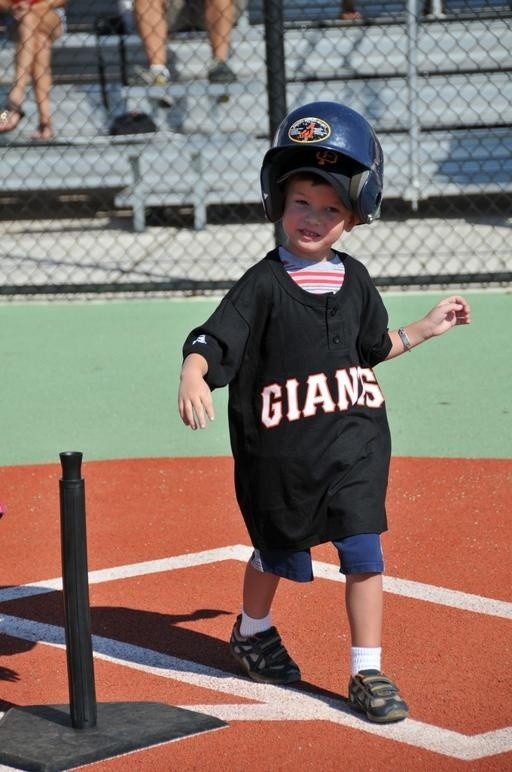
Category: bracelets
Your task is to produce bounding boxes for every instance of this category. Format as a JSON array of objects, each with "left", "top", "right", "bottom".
[{"left": 398, "top": 325, "right": 414, "bottom": 354}]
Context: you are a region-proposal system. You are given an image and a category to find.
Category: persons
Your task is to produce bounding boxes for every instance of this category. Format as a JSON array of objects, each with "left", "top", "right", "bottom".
[
  {"left": 125, "top": 0, "right": 242, "bottom": 86},
  {"left": 1, "top": 0, "right": 72, "bottom": 145},
  {"left": 172, "top": 101, "right": 472, "bottom": 728}
]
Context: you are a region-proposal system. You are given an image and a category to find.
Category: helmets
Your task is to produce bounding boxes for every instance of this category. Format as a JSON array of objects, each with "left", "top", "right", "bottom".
[{"left": 260, "top": 101, "right": 385, "bottom": 229}]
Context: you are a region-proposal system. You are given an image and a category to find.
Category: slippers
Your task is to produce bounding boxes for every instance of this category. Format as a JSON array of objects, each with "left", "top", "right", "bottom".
[
  {"left": 33, "top": 120, "right": 53, "bottom": 140},
  {"left": 0, "top": 97, "right": 25, "bottom": 131}
]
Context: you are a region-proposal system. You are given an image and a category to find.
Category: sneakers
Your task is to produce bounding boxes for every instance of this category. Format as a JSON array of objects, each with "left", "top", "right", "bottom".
[
  {"left": 348, "top": 668, "right": 407, "bottom": 724},
  {"left": 148, "top": 63, "right": 175, "bottom": 107},
  {"left": 227, "top": 614, "right": 302, "bottom": 688},
  {"left": 207, "top": 60, "right": 236, "bottom": 103}
]
